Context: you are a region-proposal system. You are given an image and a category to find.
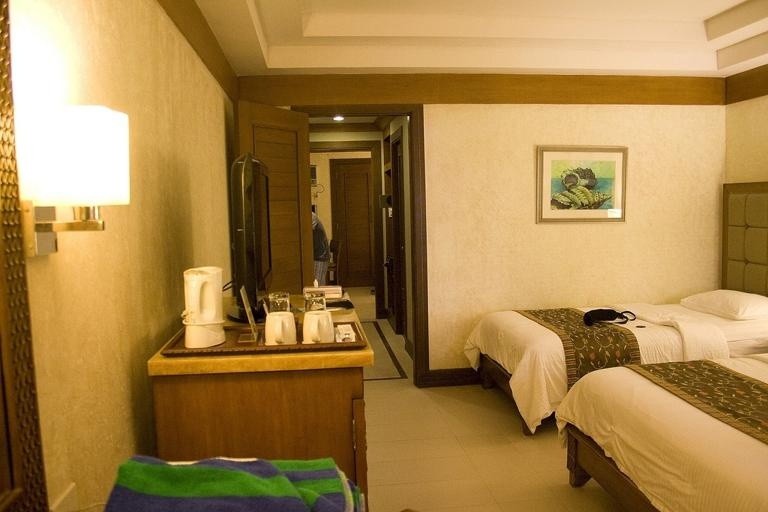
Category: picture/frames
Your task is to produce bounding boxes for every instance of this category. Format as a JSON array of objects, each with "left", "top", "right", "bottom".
[{"left": 535, "top": 143, "right": 628, "bottom": 222}]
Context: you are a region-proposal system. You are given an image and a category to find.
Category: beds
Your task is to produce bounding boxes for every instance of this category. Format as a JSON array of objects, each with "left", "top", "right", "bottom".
[
  {"left": 461, "top": 179, "right": 767, "bottom": 434},
  {"left": 553, "top": 351, "right": 767, "bottom": 510}
]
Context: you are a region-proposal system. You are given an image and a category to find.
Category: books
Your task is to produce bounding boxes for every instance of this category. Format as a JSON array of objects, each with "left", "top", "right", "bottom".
[{"left": 303, "top": 286, "right": 343, "bottom": 300}]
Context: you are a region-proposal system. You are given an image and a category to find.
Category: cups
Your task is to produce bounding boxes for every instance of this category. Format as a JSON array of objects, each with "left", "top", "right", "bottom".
[
  {"left": 302, "top": 311, "right": 335, "bottom": 345},
  {"left": 303, "top": 289, "right": 327, "bottom": 312},
  {"left": 265, "top": 312, "right": 298, "bottom": 345},
  {"left": 269, "top": 292, "right": 290, "bottom": 313}
]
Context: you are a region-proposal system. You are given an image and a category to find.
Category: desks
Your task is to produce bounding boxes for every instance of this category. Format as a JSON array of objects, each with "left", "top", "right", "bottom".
[{"left": 145, "top": 292, "right": 375, "bottom": 511}]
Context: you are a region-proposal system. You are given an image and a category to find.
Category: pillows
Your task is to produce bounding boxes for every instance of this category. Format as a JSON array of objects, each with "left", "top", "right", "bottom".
[{"left": 679, "top": 288, "right": 767, "bottom": 322}]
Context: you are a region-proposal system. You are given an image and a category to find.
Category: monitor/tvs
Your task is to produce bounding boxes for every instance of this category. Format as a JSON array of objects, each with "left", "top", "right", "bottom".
[{"left": 225, "top": 152, "right": 273, "bottom": 324}]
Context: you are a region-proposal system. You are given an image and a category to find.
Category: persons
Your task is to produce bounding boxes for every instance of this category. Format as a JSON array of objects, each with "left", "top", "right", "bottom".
[{"left": 312, "top": 210, "right": 332, "bottom": 285}]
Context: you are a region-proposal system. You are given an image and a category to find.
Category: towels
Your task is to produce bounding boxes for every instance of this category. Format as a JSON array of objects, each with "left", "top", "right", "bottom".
[{"left": 102, "top": 453, "right": 360, "bottom": 512}]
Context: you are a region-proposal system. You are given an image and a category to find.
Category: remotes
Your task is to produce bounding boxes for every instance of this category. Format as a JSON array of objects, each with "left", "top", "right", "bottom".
[{"left": 326, "top": 299, "right": 354, "bottom": 309}]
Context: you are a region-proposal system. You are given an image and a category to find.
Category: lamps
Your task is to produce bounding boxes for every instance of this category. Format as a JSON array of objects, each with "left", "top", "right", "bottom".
[{"left": 14, "top": 88, "right": 132, "bottom": 257}]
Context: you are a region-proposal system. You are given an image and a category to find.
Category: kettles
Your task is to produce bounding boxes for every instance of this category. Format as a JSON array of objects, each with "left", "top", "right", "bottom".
[{"left": 183, "top": 266, "right": 226, "bottom": 348}]
